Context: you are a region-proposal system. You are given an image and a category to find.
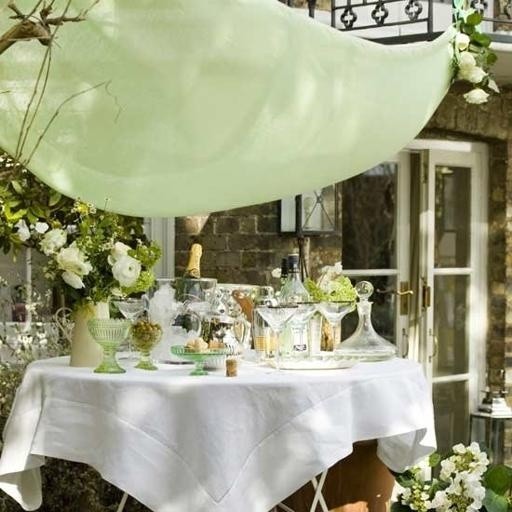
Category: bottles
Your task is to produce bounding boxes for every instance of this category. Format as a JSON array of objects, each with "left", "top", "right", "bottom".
[
  {"left": 277, "top": 253, "right": 312, "bottom": 359},
  {"left": 253, "top": 285, "right": 278, "bottom": 361},
  {"left": 173, "top": 243, "right": 206, "bottom": 335},
  {"left": 333, "top": 280, "right": 399, "bottom": 362}
]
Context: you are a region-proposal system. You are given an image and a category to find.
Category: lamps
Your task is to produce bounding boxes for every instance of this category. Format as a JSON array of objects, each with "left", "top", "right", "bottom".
[{"left": 280, "top": 182, "right": 340, "bottom": 237}]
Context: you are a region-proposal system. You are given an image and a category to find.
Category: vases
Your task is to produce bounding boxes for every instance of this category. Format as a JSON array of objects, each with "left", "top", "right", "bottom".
[{"left": 69, "top": 300, "right": 110, "bottom": 367}]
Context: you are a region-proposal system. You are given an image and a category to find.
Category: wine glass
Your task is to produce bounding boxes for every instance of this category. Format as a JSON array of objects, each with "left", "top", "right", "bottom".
[
  {"left": 87, "top": 319, "right": 132, "bottom": 373},
  {"left": 314, "top": 301, "right": 353, "bottom": 365},
  {"left": 113, "top": 300, "right": 147, "bottom": 362},
  {"left": 128, "top": 328, "right": 163, "bottom": 371},
  {"left": 257, "top": 302, "right": 320, "bottom": 376}
]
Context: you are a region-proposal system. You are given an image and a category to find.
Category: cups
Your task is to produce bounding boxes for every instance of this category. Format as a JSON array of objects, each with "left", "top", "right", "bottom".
[{"left": 148, "top": 278, "right": 259, "bottom": 364}]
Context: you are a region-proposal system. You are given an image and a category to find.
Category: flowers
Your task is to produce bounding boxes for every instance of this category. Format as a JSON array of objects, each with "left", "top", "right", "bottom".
[
  {"left": 389, "top": 442, "right": 512, "bottom": 512},
  {"left": 0, "top": 196, "right": 161, "bottom": 321}
]
